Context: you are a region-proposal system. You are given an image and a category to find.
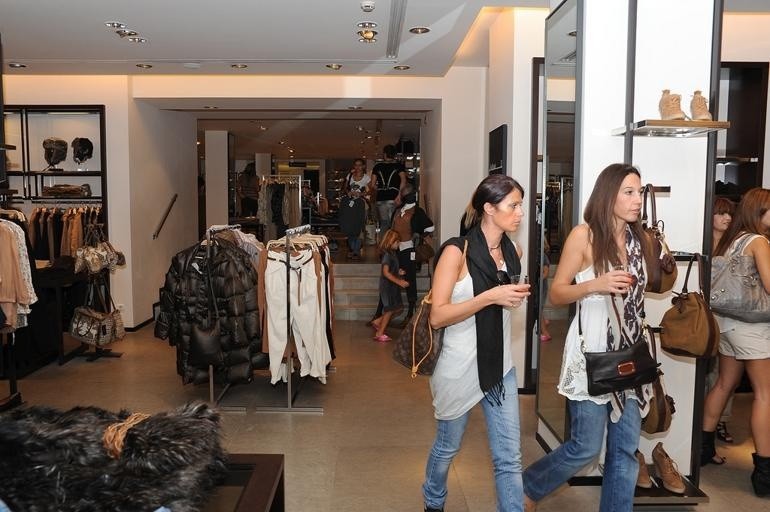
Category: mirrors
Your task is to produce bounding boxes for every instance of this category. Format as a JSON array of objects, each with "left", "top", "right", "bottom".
[{"left": 536, "top": 0, "right": 584, "bottom": 444}]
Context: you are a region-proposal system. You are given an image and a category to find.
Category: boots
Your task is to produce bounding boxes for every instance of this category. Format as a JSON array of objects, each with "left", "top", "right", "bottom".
[
  {"left": 751, "top": 453, "right": 770, "bottom": 498},
  {"left": 702, "top": 431, "right": 725, "bottom": 466}
]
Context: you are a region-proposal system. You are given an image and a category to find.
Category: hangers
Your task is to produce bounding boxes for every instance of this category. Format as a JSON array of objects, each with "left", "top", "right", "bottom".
[
  {"left": 1, "top": 201, "right": 23, "bottom": 231},
  {"left": 201, "top": 223, "right": 240, "bottom": 251},
  {"left": 267, "top": 225, "right": 327, "bottom": 258},
  {"left": 260, "top": 174, "right": 322, "bottom": 207},
  {"left": 30, "top": 200, "right": 101, "bottom": 217},
  {"left": 546, "top": 181, "right": 561, "bottom": 193}
]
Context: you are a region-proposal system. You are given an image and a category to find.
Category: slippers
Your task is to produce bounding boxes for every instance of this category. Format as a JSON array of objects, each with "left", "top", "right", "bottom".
[{"left": 374, "top": 334, "right": 392, "bottom": 342}]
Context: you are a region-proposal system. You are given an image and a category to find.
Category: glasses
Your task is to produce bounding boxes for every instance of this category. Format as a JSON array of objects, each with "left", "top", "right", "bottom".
[{"left": 497, "top": 270, "right": 511, "bottom": 286}]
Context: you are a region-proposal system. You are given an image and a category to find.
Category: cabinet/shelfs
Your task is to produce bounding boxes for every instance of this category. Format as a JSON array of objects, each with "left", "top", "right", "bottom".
[
  {"left": 598, "top": 119, "right": 729, "bottom": 511},
  {"left": 4, "top": 110, "right": 102, "bottom": 199}
]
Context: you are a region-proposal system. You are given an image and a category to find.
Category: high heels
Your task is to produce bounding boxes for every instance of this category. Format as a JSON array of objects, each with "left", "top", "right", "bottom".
[{"left": 635, "top": 442, "right": 686, "bottom": 493}]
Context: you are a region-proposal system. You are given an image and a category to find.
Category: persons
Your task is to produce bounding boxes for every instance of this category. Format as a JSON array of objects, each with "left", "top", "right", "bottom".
[
  {"left": 366, "top": 184, "right": 434, "bottom": 329},
  {"left": 301, "top": 180, "right": 311, "bottom": 207},
  {"left": 372, "top": 144, "right": 406, "bottom": 244},
  {"left": 422, "top": 174, "right": 525, "bottom": 512},
  {"left": 522, "top": 164, "right": 653, "bottom": 512},
  {"left": 372, "top": 230, "right": 408, "bottom": 342},
  {"left": 699, "top": 187, "right": 770, "bottom": 498},
  {"left": 712, "top": 197, "right": 735, "bottom": 443},
  {"left": 342, "top": 159, "right": 372, "bottom": 260},
  {"left": 236, "top": 162, "right": 261, "bottom": 218}
]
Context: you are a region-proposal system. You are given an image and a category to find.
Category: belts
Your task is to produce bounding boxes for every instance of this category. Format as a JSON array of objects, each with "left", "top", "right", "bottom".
[{"left": 267, "top": 254, "right": 313, "bottom": 305}]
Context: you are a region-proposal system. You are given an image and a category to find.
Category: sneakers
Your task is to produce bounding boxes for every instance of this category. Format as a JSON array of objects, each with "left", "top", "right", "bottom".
[{"left": 659, "top": 90, "right": 712, "bottom": 120}]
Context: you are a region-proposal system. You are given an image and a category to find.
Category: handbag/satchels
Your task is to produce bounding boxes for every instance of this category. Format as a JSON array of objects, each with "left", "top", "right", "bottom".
[
  {"left": 74, "top": 227, "right": 126, "bottom": 274},
  {"left": 187, "top": 320, "right": 228, "bottom": 365},
  {"left": 70, "top": 277, "right": 125, "bottom": 346},
  {"left": 393, "top": 232, "right": 469, "bottom": 378},
  {"left": 584, "top": 324, "right": 675, "bottom": 434},
  {"left": 642, "top": 184, "right": 770, "bottom": 358}
]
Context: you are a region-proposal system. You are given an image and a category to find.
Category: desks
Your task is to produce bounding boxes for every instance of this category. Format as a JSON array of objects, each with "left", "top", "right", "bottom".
[{"left": 204, "top": 453, "right": 285, "bottom": 512}]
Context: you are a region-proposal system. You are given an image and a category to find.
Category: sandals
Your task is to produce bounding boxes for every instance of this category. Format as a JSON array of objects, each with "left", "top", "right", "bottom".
[{"left": 717, "top": 421, "right": 733, "bottom": 442}]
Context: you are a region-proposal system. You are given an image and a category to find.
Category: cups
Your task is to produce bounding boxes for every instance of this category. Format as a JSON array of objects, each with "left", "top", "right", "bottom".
[
  {"left": 510, "top": 275, "right": 528, "bottom": 299},
  {"left": 613, "top": 265, "right": 630, "bottom": 289}
]
